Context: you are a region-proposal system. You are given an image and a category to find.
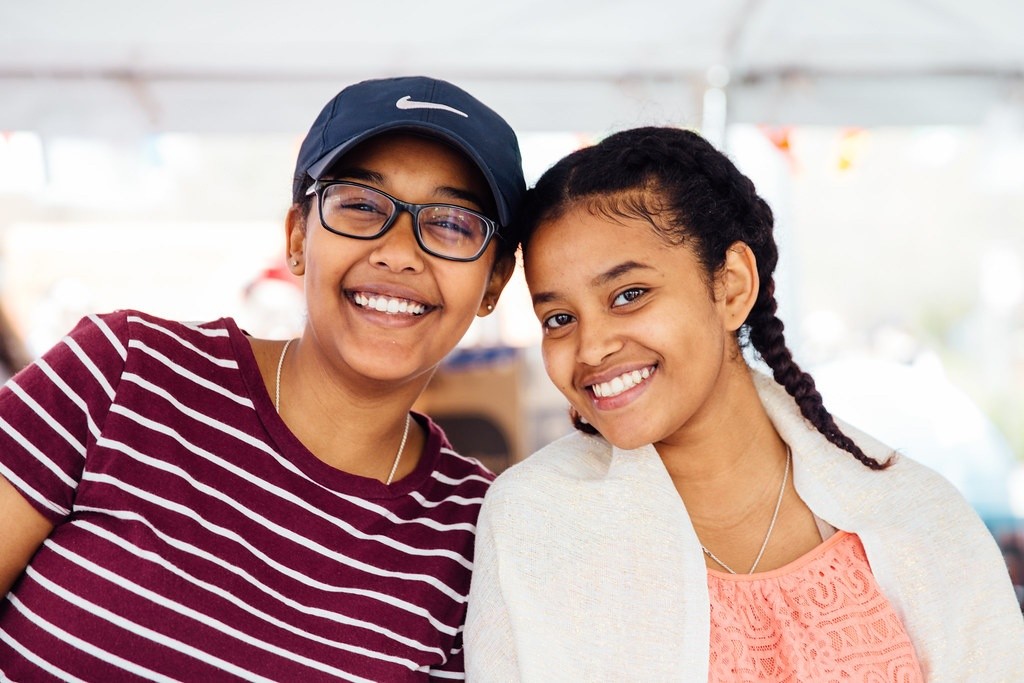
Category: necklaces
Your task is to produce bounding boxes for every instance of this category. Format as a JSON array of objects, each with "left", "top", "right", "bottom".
[
  {"left": 701, "top": 449, "right": 790, "bottom": 577},
  {"left": 274, "top": 334, "right": 411, "bottom": 486}
]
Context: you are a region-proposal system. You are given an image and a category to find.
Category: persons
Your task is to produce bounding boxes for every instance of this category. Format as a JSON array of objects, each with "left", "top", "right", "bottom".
[
  {"left": 461, "top": 128, "right": 1017, "bottom": 683},
  {"left": 0, "top": 76, "right": 517, "bottom": 683}
]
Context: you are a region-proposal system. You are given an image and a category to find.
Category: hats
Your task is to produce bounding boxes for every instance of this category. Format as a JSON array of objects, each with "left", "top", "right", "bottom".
[{"left": 293, "top": 75, "right": 526, "bottom": 254}]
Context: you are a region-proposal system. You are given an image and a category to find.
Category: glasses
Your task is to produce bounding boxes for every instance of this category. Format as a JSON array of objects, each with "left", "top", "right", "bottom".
[{"left": 305, "top": 179, "right": 504, "bottom": 262}]
